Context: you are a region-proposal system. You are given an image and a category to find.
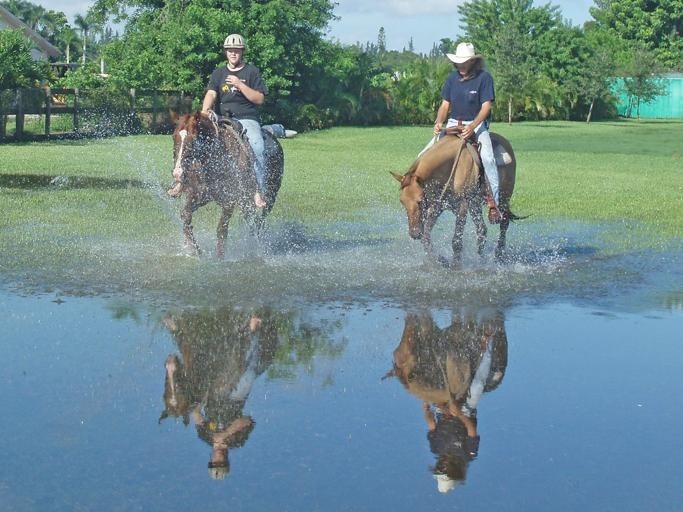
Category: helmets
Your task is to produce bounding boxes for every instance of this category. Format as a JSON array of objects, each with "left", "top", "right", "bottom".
[{"left": 223, "top": 34, "right": 245, "bottom": 49}]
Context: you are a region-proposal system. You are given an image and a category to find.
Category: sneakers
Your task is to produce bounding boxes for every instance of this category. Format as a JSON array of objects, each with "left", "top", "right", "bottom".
[{"left": 488, "top": 207, "right": 501, "bottom": 224}]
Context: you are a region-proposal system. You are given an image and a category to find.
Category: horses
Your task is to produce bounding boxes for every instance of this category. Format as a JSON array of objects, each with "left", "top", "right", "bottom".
[
  {"left": 386, "top": 130, "right": 531, "bottom": 267},
  {"left": 381, "top": 302, "right": 509, "bottom": 408},
  {"left": 153, "top": 311, "right": 281, "bottom": 423},
  {"left": 166, "top": 109, "right": 286, "bottom": 257}
]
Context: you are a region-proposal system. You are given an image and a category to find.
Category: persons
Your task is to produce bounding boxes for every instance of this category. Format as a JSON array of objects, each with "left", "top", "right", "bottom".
[
  {"left": 424, "top": 336, "right": 495, "bottom": 492},
  {"left": 419, "top": 42, "right": 501, "bottom": 224},
  {"left": 201, "top": 33, "right": 267, "bottom": 208},
  {"left": 193, "top": 318, "right": 263, "bottom": 481}
]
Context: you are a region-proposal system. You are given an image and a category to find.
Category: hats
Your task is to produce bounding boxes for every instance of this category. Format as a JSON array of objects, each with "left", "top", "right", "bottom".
[{"left": 445, "top": 42, "right": 484, "bottom": 68}]
